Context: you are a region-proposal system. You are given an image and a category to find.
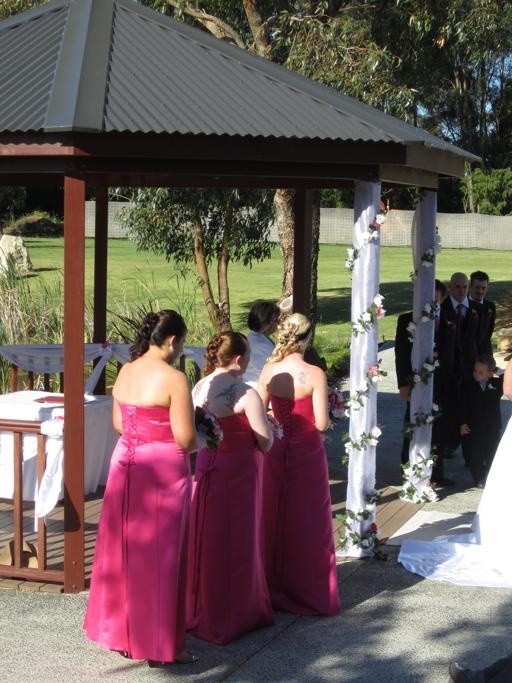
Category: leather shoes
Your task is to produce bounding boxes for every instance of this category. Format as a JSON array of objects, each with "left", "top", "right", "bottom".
[{"left": 475, "top": 477, "right": 484, "bottom": 489}]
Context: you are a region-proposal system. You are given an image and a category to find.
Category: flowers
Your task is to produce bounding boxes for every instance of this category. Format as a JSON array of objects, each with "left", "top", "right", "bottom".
[
  {"left": 327, "top": 391, "right": 350, "bottom": 421},
  {"left": 399, "top": 224, "right": 441, "bottom": 498},
  {"left": 264, "top": 412, "right": 284, "bottom": 439},
  {"left": 192, "top": 403, "right": 224, "bottom": 448},
  {"left": 338, "top": 200, "right": 388, "bottom": 557}
]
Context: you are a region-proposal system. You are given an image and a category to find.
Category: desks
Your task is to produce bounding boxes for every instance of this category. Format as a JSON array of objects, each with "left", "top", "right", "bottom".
[{"left": 2, "top": 390, "right": 112, "bottom": 501}]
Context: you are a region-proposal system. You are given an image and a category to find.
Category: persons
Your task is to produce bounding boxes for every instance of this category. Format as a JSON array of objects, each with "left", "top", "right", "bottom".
[
  {"left": 471, "top": 322, "right": 512, "bottom": 590},
  {"left": 191, "top": 327, "right": 277, "bottom": 644},
  {"left": 83, "top": 308, "right": 226, "bottom": 668},
  {"left": 441, "top": 271, "right": 480, "bottom": 462},
  {"left": 396, "top": 276, "right": 456, "bottom": 489},
  {"left": 467, "top": 268, "right": 497, "bottom": 360},
  {"left": 241, "top": 298, "right": 281, "bottom": 385},
  {"left": 256, "top": 310, "right": 341, "bottom": 618},
  {"left": 458, "top": 352, "right": 505, "bottom": 489}
]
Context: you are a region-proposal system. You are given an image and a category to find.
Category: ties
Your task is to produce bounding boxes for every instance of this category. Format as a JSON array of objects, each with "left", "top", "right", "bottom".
[{"left": 456, "top": 303, "right": 464, "bottom": 323}]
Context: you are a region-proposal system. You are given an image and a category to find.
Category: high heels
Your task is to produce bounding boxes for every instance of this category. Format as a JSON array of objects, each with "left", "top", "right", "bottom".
[{"left": 147, "top": 651, "right": 200, "bottom": 668}]
